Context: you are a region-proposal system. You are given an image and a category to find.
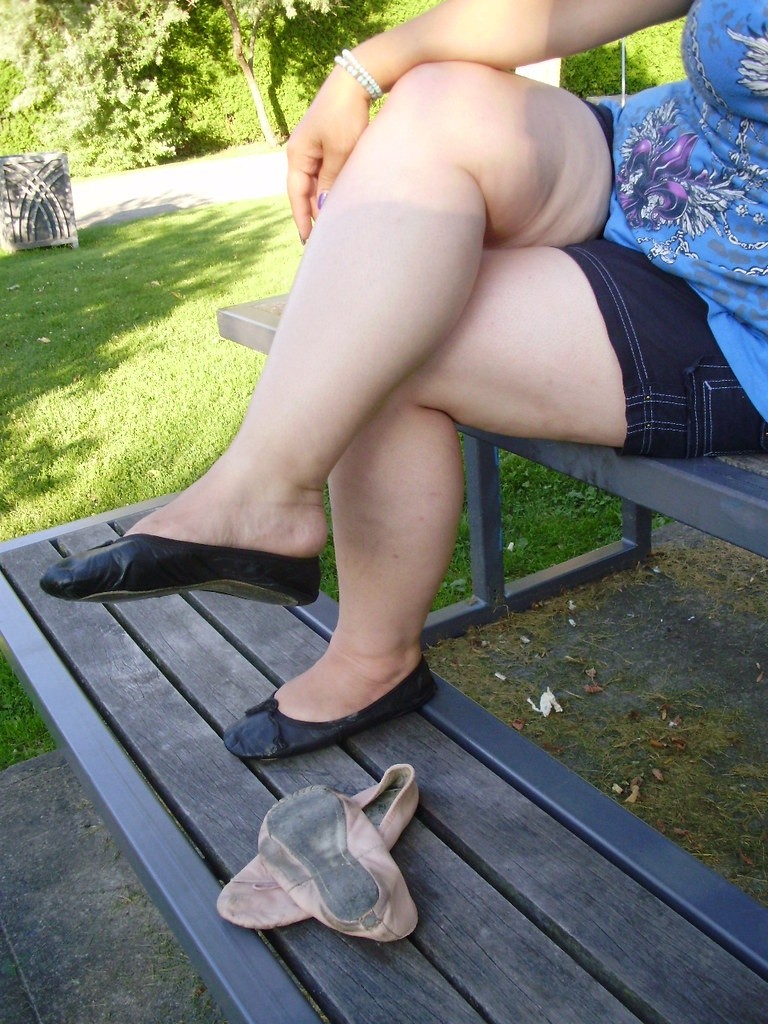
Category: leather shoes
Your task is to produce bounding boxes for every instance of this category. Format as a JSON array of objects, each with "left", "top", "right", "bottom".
[
  {"left": 223, "top": 653, "right": 439, "bottom": 760},
  {"left": 39, "top": 533, "right": 322, "bottom": 606}
]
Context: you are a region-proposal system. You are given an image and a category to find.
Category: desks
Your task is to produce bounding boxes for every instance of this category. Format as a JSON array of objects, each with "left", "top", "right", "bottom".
[{"left": 215, "top": 291, "right": 768, "bottom": 649}]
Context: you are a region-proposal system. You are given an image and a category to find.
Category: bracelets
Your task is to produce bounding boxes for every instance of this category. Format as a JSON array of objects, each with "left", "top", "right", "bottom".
[
  {"left": 332, "top": 56, "right": 381, "bottom": 103},
  {"left": 341, "top": 50, "right": 384, "bottom": 97}
]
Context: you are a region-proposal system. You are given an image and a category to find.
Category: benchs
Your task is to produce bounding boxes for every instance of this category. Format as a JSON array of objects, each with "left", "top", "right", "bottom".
[{"left": 0, "top": 487, "right": 766, "bottom": 1024}]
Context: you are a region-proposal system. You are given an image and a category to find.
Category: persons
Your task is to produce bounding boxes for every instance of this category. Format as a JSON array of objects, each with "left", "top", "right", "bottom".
[{"left": 34, "top": 2, "right": 768, "bottom": 762}]
